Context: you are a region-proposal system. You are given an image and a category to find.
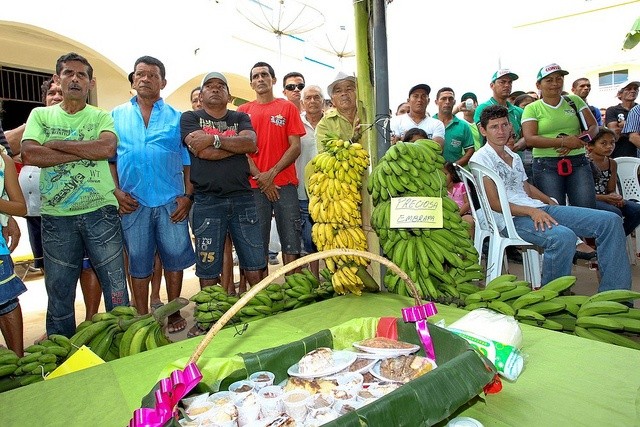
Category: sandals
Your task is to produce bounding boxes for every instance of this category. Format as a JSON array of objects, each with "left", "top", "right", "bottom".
[{"left": 187, "top": 322, "right": 208, "bottom": 338}]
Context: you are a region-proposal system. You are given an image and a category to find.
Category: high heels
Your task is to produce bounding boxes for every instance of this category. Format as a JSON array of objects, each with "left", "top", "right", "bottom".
[{"left": 572, "top": 250, "right": 597, "bottom": 264}]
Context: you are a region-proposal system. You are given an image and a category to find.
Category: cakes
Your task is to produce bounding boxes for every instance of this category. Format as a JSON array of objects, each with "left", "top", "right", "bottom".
[{"left": 181, "top": 335, "right": 434, "bottom": 427}]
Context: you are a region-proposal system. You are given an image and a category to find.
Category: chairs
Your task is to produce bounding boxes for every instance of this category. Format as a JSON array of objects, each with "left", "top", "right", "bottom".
[
  {"left": 614, "top": 156, "right": 640, "bottom": 258},
  {"left": 468, "top": 161, "right": 542, "bottom": 290},
  {"left": 454, "top": 164, "right": 511, "bottom": 282}
]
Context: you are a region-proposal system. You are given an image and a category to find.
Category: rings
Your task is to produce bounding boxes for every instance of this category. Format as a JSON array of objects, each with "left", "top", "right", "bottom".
[{"left": 188, "top": 145, "right": 192, "bottom": 148}]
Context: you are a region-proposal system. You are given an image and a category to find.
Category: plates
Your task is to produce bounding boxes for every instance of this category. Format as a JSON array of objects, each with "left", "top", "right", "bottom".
[
  {"left": 322, "top": 358, "right": 380, "bottom": 381},
  {"left": 362, "top": 380, "right": 381, "bottom": 388},
  {"left": 369, "top": 355, "right": 438, "bottom": 383},
  {"left": 286, "top": 350, "right": 358, "bottom": 378},
  {"left": 351, "top": 339, "right": 422, "bottom": 354},
  {"left": 446, "top": 416, "right": 484, "bottom": 427},
  {"left": 343, "top": 347, "right": 401, "bottom": 360}
]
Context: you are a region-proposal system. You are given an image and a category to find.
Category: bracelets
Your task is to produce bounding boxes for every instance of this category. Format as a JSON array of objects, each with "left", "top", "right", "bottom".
[
  {"left": 453, "top": 162, "right": 457, "bottom": 170},
  {"left": 184, "top": 194, "right": 194, "bottom": 202},
  {"left": 562, "top": 136, "right": 564, "bottom": 149}
]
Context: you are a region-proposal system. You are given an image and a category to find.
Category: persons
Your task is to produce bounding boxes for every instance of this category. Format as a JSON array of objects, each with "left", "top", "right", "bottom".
[
  {"left": 268, "top": 72, "right": 305, "bottom": 266},
  {"left": 236, "top": 62, "right": 306, "bottom": 280},
  {"left": 17, "top": 77, "right": 102, "bottom": 320},
  {"left": 108, "top": 56, "right": 197, "bottom": 333},
  {"left": 20, "top": 53, "right": 130, "bottom": 339},
  {"left": 599, "top": 108, "right": 606, "bottom": 125},
  {"left": 588, "top": 128, "right": 640, "bottom": 236},
  {"left": 390, "top": 83, "right": 445, "bottom": 150},
  {"left": 188, "top": 87, "right": 235, "bottom": 296},
  {"left": 315, "top": 71, "right": 361, "bottom": 155},
  {"left": 473, "top": 69, "right": 524, "bottom": 163},
  {"left": 432, "top": 87, "right": 475, "bottom": 181},
  {"left": 468, "top": 105, "right": 634, "bottom": 308},
  {"left": 605, "top": 79, "right": 640, "bottom": 158},
  {"left": 403, "top": 128, "right": 428, "bottom": 142},
  {"left": 395, "top": 102, "right": 411, "bottom": 116},
  {"left": 506, "top": 129, "right": 598, "bottom": 265},
  {"left": 180, "top": 71, "right": 267, "bottom": 338},
  {"left": 0, "top": 126, "right": 27, "bottom": 359},
  {"left": 508, "top": 91, "right": 540, "bottom": 109},
  {"left": 571, "top": 78, "right": 602, "bottom": 236},
  {"left": 621, "top": 104, "right": 640, "bottom": 158},
  {"left": 295, "top": 84, "right": 325, "bottom": 280},
  {"left": 452, "top": 92, "right": 481, "bottom": 172},
  {"left": 520, "top": 63, "right": 599, "bottom": 208},
  {"left": 443, "top": 161, "right": 475, "bottom": 240}
]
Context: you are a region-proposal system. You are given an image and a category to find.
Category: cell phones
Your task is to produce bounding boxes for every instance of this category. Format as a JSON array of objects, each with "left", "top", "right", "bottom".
[{"left": 579, "top": 134, "right": 593, "bottom": 144}]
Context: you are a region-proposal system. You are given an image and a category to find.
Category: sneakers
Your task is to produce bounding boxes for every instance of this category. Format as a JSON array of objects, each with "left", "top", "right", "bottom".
[
  {"left": 507, "top": 251, "right": 524, "bottom": 264},
  {"left": 268, "top": 256, "right": 279, "bottom": 265}
]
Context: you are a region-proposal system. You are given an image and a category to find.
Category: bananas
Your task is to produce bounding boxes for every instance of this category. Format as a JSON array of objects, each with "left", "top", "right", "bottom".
[
  {"left": 307, "top": 131, "right": 371, "bottom": 293},
  {"left": 463, "top": 271, "right": 640, "bottom": 353},
  {"left": 189, "top": 267, "right": 333, "bottom": 334},
  {"left": 1, "top": 305, "right": 185, "bottom": 394},
  {"left": 365, "top": 139, "right": 481, "bottom": 349}
]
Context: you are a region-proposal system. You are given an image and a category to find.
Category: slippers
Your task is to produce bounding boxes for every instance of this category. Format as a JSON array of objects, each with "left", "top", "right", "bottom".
[
  {"left": 168, "top": 318, "right": 185, "bottom": 334},
  {"left": 150, "top": 303, "right": 164, "bottom": 309}
]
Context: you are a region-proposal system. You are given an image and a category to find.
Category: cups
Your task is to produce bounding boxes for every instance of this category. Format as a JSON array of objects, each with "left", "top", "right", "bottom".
[
  {"left": 207, "top": 390, "right": 235, "bottom": 408},
  {"left": 182, "top": 391, "right": 211, "bottom": 409},
  {"left": 257, "top": 386, "right": 284, "bottom": 418},
  {"left": 228, "top": 380, "right": 255, "bottom": 407},
  {"left": 304, "top": 393, "right": 334, "bottom": 411},
  {"left": 233, "top": 397, "right": 261, "bottom": 426},
  {"left": 186, "top": 402, "right": 215, "bottom": 420},
  {"left": 305, "top": 407, "right": 339, "bottom": 427},
  {"left": 356, "top": 391, "right": 378, "bottom": 404},
  {"left": 282, "top": 389, "right": 310, "bottom": 418},
  {"left": 333, "top": 401, "right": 365, "bottom": 416},
  {"left": 250, "top": 371, "right": 276, "bottom": 391},
  {"left": 208, "top": 407, "right": 239, "bottom": 426},
  {"left": 330, "top": 386, "right": 356, "bottom": 403},
  {"left": 337, "top": 372, "right": 364, "bottom": 387}
]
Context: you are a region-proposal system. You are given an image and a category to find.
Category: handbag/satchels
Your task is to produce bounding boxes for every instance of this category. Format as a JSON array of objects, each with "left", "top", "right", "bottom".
[{"left": 589, "top": 159, "right": 602, "bottom": 178}]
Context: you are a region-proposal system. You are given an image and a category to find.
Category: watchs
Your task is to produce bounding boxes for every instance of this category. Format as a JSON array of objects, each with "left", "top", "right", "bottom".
[{"left": 214, "top": 134, "right": 221, "bottom": 149}]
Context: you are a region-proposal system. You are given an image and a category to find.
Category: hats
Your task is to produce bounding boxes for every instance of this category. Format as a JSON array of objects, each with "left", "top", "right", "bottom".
[
  {"left": 327, "top": 71, "right": 356, "bottom": 97},
  {"left": 618, "top": 80, "right": 640, "bottom": 92},
  {"left": 201, "top": 71, "right": 227, "bottom": 90},
  {"left": 537, "top": 63, "right": 569, "bottom": 82},
  {"left": 461, "top": 92, "right": 477, "bottom": 102},
  {"left": 409, "top": 84, "right": 430, "bottom": 98},
  {"left": 491, "top": 70, "right": 518, "bottom": 82}
]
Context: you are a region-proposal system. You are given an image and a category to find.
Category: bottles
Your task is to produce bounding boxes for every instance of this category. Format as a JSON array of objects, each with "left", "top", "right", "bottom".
[{"left": 465, "top": 99, "right": 473, "bottom": 112}]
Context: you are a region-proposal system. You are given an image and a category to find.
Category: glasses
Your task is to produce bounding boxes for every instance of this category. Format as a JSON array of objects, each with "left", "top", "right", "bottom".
[{"left": 284, "top": 83, "right": 305, "bottom": 90}]
